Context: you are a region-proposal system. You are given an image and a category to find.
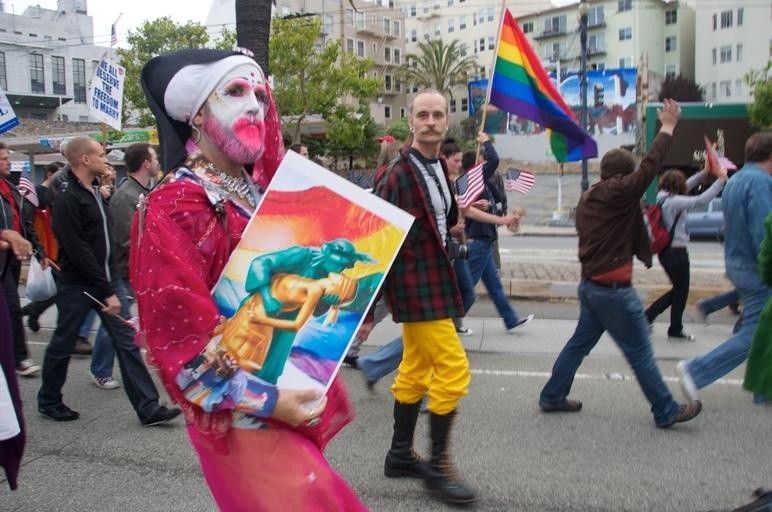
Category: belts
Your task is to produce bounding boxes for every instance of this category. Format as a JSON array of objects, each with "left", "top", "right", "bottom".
[{"left": 591, "top": 280, "right": 632, "bottom": 288}]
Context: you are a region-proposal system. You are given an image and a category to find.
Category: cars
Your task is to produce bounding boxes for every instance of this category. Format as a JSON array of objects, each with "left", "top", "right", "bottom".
[{"left": 679, "top": 199, "right": 730, "bottom": 243}]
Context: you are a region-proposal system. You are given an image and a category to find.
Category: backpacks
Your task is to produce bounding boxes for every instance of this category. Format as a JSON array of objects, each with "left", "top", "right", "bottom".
[{"left": 642, "top": 196, "right": 681, "bottom": 253}]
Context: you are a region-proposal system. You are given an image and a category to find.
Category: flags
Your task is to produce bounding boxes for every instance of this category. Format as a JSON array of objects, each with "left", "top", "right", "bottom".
[{"left": 487, "top": 5, "right": 600, "bottom": 165}]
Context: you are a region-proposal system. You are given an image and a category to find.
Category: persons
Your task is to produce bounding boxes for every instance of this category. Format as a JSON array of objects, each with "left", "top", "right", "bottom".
[
  {"left": 211, "top": 235, "right": 374, "bottom": 385},
  {"left": 674, "top": 131, "right": 770, "bottom": 407},
  {"left": 373, "top": 89, "right": 478, "bottom": 504},
  {"left": 693, "top": 287, "right": 740, "bottom": 326},
  {"left": 128, "top": 46, "right": 372, "bottom": 512},
  {"left": 644, "top": 141, "right": 727, "bottom": 342},
  {"left": 0, "top": 135, "right": 182, "bottom": 490},
  {"left": 742, "top": 213, "right": 771, "bottom": 405},
  {"left": 537, "top": 98, "right": 703, "bottom": 430},
  {"left": 290, "top": 128, "right": 534, "bottom": 412}
]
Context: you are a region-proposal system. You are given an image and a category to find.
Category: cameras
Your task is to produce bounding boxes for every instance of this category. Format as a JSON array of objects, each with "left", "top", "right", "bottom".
[{"left": 446, "top": 236, "right": 468, "bottom": 260}]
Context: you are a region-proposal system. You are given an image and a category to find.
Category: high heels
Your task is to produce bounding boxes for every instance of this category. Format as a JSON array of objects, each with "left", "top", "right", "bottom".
[{"left": 21, "top": 306, "right": 40, "bottom": 331}]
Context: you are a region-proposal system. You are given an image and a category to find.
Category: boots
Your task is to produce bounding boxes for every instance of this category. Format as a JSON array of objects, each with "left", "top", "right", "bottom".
[
  {"left": 383, "top": 397, "right": 428, "bottom": 478},
  {"left": 420, "top": 411, "right": 475, "bottom": 505}
]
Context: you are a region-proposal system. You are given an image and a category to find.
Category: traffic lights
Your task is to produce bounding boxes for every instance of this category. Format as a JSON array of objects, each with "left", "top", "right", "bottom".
[{"left": 591, "top": 82, "right": 605, "bottom": 108}]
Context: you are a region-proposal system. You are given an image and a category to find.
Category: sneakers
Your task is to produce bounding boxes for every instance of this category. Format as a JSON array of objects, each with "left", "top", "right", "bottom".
[
  {"left": 14, "top": 358, "right": 44, "bottom": 376},
  {"left": 38, "top": 400, "right": 80, "bottom": 421},
  {"left": 658, "top": 400, "right": 702, "bottom": 429},
  {"left": 87, "top": 364, "right": 120, "bottom": 389},
  {"left": 455, "top": 325, "right": 474, "bottom": 337},
  {"left": 538, "top": 399, "right": 582, "bottom": 412},
  {"left": 506, "top": 313, "right": 535, "bottom": 333},
  {"left": 144, "top": 402, "right": 183, "bottom": 427},
  {"left": 70, "top": 336, "right": 96, "bottom": 355},
  {"left": 695, "top": 298, "right": 708, "bottom": 325},
  {"left": 676, "top": 359, "right": 700, "bottom": 402},
  {"left": 666, "top": 331, "right": 697, "bottom": 344}
]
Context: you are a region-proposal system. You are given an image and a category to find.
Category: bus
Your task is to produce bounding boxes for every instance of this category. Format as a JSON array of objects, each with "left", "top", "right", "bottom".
[{"left": 637, "top": 97, "right": 759, "bottom": 213}]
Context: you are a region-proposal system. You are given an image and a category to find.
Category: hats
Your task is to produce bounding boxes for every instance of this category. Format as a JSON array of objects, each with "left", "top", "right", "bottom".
[{"left": 142, "top": 49, "right": 267, "bottom": 174}]
